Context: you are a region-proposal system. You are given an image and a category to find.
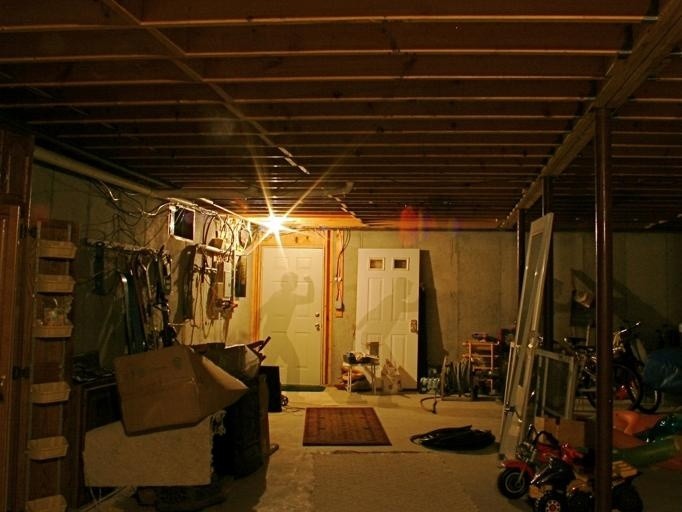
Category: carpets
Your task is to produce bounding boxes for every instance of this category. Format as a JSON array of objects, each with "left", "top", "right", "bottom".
[
  {"left": 311, "top": 450, "right": 480, "bottom": 512},
  {"left": 303, "top": 407, "right": 391, "bottom": 446}
]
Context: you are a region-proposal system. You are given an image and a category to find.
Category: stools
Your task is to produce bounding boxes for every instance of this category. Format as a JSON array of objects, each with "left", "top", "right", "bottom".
[{"left": 473, "top": 376, "right": 498, "bottom": 396}]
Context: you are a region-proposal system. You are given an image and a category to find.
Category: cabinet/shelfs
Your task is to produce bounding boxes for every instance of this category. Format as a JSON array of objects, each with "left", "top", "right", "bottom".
[
  {"left": 24, "top": 220, "right": 76, "bottom": 512},
  {"left": 462, "top": 341, "right": 499, "bottom": 391}
]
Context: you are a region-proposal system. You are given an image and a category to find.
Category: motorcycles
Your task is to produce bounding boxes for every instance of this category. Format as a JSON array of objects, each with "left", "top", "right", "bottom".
[{"left": 563, "top": 320, "right": 663, "bottom": 415}]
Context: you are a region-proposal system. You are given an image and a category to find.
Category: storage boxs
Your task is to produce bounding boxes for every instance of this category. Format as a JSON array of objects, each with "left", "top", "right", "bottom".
[{"left": 114, "top": 344, "right": 249, "bottom": 437}]
